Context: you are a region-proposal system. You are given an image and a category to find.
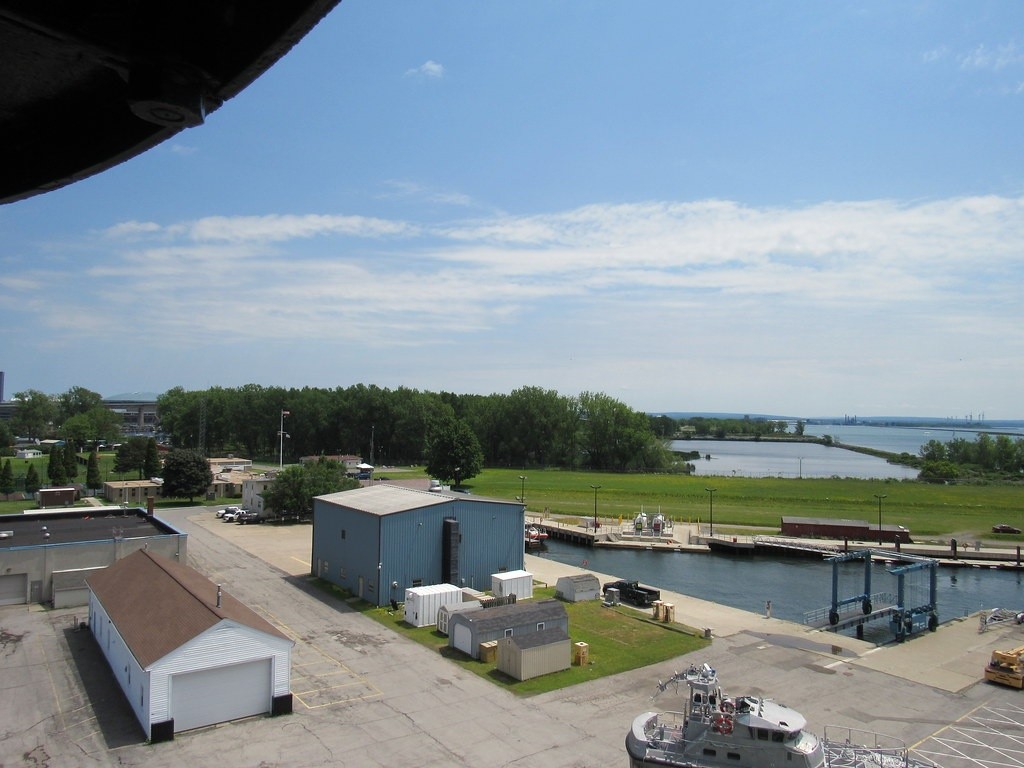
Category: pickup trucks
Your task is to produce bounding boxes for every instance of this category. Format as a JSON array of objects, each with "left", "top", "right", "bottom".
[
  {"left": 216, "top": 506, "right": 258, "bottom": 522},
  {"left": 603, "top": 580, "right": 660, "bottom": 607}
]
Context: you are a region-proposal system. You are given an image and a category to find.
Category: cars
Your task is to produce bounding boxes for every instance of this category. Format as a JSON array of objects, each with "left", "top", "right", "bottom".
[
  {"left": 237, "top": 514, "right": 265, "bottom": 525},
  {"left": 374, "top": 477, "right": 390, "bottom": 480},
  {"left": 355, "top": 473, "right": 370, "bottom": 480},
  {"left": 453, "top": 489, "right": 470, "bottom": 495},
  {"left": 992, "top": 525, "right": 1022, "bottom": 534}
]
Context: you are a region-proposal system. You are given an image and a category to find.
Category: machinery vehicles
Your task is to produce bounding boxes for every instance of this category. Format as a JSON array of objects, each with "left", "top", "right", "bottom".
[{"left": 984, "top": 646, "right": 1024, "bottom": 689}]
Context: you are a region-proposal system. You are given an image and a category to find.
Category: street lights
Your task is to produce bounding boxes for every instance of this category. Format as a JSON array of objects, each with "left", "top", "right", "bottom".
[
  {"left": 874, "top": 494, "right": 887, "bottom": 545},
  {"left": 705, "top": 488, "right": 717, "bottom": 536},
  {"left": 591, "top": 485, "right": 601, "bottom": 533},
  {"left": 280, "top": 409, "right": 290, "bottom": 469},
  {"left": 518, "top": 476, "right": 527, "bottom": 503},
  {"left": 798, "top": 457, "right": 802, "bottom": 477}
]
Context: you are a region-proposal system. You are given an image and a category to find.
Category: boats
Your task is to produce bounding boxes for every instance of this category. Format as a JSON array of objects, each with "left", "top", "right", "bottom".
[
  {"left": 525, "top": 527, "right": 548, "bottom": 544},
  {"left": 625, "top": 663, "right": 938, "bottom": 768}
]
